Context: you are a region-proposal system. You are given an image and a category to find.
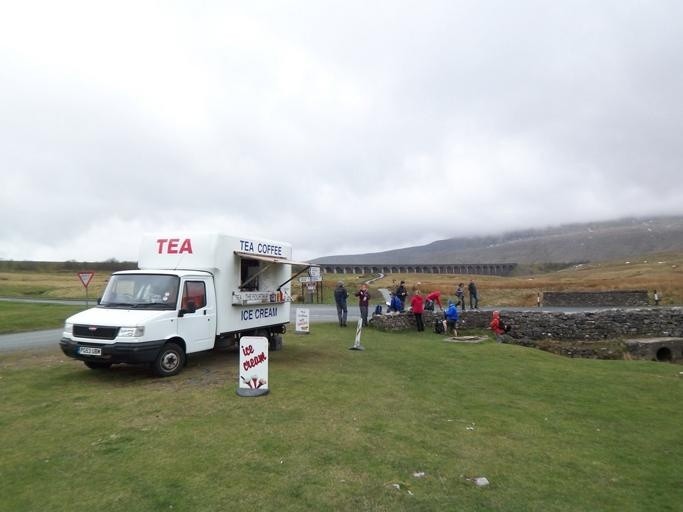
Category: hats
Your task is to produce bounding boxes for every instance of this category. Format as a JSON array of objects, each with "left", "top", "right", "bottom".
[{"left": 337, "top": 280, "right": 344, "bottom": 286}]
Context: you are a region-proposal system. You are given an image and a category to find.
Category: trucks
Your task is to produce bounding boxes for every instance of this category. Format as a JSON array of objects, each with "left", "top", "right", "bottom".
[{"left": 58, "top": 230, "right": 321, "bottom": 379}]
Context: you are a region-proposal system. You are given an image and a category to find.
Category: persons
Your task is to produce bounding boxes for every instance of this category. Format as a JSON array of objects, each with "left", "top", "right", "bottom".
[
  {"left": 396, "top": 281, "right": 409, "bottom": 309},
  {"left": 424, "top": 291, "right": 443, "bottom": 312},
  {"left": 490, "top": 310, "right": 507, "bottom": 344},
  {"left": 354, "top": 284, "right": 371, "bottom": 327},
  {"left": 455, "top": 283, "right": 467, "bottom": 310},
  {"left": 387, "top": 292, "right": 401, "bottom": 312},
  {"left": 467, "top": 280, "right": 479, "bottom": 309},
  {"left": 334, "top": 281, "right": 350, "bottom": 327},
  {"left": 441, "top": 300, "right": 459, "bottom": 337},
  {"left": 411, "top": 290, "right": 425, "bottom": 332}
]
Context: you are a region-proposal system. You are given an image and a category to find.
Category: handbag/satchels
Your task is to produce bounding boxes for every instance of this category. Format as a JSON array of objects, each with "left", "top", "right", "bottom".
[{"left": 455, "top": 289, "right": 461, "bottom": 296}]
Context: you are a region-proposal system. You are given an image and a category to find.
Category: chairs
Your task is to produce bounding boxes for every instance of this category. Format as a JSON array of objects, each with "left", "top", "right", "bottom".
[{"left": 170, "top": 283, "right": 204, "bottom": 310}]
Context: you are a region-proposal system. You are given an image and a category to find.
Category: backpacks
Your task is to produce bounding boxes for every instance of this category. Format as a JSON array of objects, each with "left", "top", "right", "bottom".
[{"left": 498, "top": 318, "right": 506, "bottom": 329}]
